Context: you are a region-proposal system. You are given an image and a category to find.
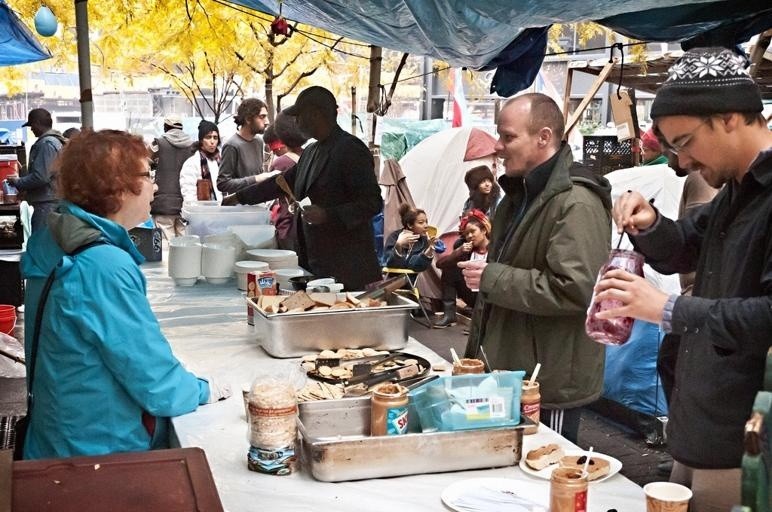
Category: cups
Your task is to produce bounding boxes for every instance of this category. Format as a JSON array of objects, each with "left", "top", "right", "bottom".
[
  {"left": 2, "top": 179, "right": 17, "bottom": 195},
  {"left": 469, "top": 259, "right": 485, "bottom": 292},
  {"left": 519, "top": 449, "right": 622, "bottom": 485},
  {"left": 0, "top": 103, "right": 26, "bottom": 147},
  {"left": 197, "top": 180, "right": 211, "bottom": 200},
  {"left": 643, "top": 482, "right": 694, "bottom": 511}
]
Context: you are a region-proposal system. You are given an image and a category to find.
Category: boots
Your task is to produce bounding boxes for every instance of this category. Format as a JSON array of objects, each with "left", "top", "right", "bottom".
[{"left": 432, "top": 299, "right": 460, "bottom": 331}]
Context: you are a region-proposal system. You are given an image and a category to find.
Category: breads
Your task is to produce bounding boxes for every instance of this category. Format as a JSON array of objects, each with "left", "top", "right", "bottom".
[
  {"left": 525, "top": 443, "right": 563, "bottom": 471},
  {"left": 257, "top": 289, "right": 388, "bottom": 314},
  {"left": 559, "top": 455, "right": 611, "bottom": 482}
]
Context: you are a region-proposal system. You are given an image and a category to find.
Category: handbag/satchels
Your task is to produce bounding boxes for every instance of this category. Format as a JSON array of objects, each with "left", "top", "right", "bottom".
[{"left": 11, "top": 392, "right": 160, "bottom": 467}]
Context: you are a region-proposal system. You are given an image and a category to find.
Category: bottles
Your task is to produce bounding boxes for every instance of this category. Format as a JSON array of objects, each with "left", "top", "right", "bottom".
[
  {"left": 521, "top": 381, "right": 543, "bottom": 434},
  {"left": 452, "top": 359, "right": 484, "bottom": 376},
  {"left": 742, "top": 430, "right": 770, "bottom": 510}
]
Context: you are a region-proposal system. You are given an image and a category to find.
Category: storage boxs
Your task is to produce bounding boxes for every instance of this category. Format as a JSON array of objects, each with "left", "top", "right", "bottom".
[
  {"left": 181, "top": 198, "right": 268, "bottom": 236},
  {"left": 0, "top": 154, "right": 20, "bottom": 192}
]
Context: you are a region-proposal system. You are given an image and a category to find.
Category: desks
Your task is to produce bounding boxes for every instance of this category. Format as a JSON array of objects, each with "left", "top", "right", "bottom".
[{"left": 137, "top": 238, "right": 647, "bottom": 511}]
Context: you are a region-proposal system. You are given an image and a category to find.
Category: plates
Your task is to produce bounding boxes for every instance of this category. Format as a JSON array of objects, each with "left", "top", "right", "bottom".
[
  {"left": 169, "top": 227, "right": 341, "bottom": 292},
  {"left": 439, "top": 477, "right": 548, "bottom": 511}
]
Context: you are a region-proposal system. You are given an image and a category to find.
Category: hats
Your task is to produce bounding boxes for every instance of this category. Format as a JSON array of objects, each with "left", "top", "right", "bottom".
[
  {"left": 648, "top": 45, "right": 764, "bottom": 119},
  {"left": 282, "top": 84, "right": 340, "bottom": 117},
  {"left": 463, "top": 162, "right": 493, "bottom": 188},
  {"left": 161, "top": 115, "right": 222, "bottom": 145},
  {"left": 20, "top": 108, "right": 50, "bottom": 126}
]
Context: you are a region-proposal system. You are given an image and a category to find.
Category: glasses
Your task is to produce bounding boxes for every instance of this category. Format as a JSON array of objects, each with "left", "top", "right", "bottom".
[
  {"left": 130, "top": 164, "right": 159, "bottom": 182},
  {"left": 661, "top": 120, "right": 712, "bottom": 157}
]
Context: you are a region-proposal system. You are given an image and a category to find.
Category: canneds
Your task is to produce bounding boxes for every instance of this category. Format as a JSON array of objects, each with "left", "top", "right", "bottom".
[
  {"left": 452, "top": 359, "right": 485, "bottom": 386},
  {"left": 519, "top": 380, "right": 541, "bottom": 435}
]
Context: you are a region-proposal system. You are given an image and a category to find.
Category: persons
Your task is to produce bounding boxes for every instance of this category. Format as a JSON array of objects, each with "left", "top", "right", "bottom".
[
  {"left": 594, "top": 46, "right": 772, "bottom": 511},
  {"left": 649, "top": 121, "right": 715, "bottom": 410},
  {"left": 456, "top": 92, "right": 618, "bottom": 448},
  {"left": 152, "top": 84, "right": 501, "bottom": 328},
  {"left": 19, "top": 130, "right": 233, "bottom": 460},
  {"left": 640, "top": 125, "right": 667, "bottom": 164},
  {"left": 9, "top": 108, "right": 63, "bottom": 231}
]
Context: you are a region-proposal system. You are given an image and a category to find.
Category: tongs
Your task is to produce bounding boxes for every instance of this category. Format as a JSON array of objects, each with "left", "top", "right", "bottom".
[
  {"left": 355, "top": 273, "right": 410, "bottom": 301},
  {"left": 319, "top": 352, "right": 437, "bottom": 391}
]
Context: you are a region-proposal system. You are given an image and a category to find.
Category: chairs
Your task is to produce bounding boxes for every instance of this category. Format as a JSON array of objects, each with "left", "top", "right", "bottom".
[{"left": 382, "top": 225, "right": 438, "bottom": 329}]
